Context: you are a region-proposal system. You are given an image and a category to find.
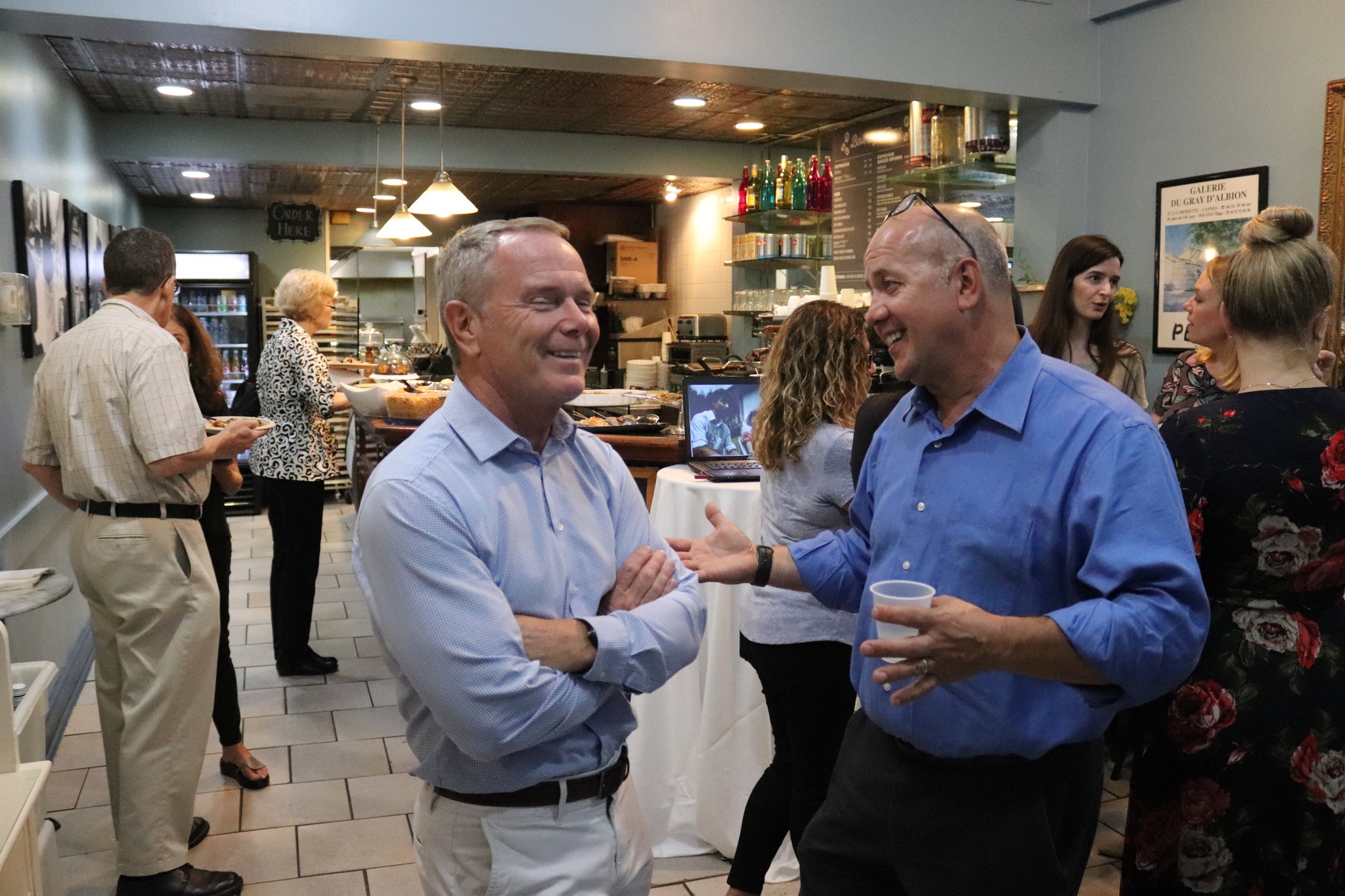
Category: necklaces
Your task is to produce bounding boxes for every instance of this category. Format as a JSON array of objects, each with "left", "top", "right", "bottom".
[{"left": 1239, "top": 376, "right": 1317, "bottom": 392}]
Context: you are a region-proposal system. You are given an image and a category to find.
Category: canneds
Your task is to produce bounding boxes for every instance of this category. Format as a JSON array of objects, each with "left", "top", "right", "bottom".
[
  {"left": 216, "top": 295, "right": 247, "bottom": 312},
  {"left": 964, "top": 106, "right": 1010, "bottom": 156},
  {"left": 909, "top": 101, "right": 940, "bottom": 167},
  {"left": 757, "top": 233, "right": 832, "bottom": 259}
]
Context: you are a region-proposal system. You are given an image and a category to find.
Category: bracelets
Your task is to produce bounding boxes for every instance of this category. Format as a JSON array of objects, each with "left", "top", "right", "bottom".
[
  {"left": 578, "top": 619, "right": 598, "bottom": 650},
  {"left": 750, "top": 544, "right": 774, "bottom": 588}
]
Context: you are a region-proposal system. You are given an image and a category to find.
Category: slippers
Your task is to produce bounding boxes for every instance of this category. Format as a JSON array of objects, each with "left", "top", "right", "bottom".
[{"left": 219, "top": 755, "right": 270, "bottom": 789}]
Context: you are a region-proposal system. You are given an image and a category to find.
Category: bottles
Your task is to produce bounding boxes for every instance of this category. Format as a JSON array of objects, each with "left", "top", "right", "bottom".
[
  {"left": 820, "top": 156, "right": 833, "bottom": 211},
  {"left": 807, "top": 155, "right": 821, "bottom": 210},
  {"left": 792, "top": 158, "right": 807, "bottom": 210},
  {"left": 199, "top": 317, "right": 240, "bottom": 344},
  {"left": 13, "top": 683, "right": 27, "bottom": 711},
  {"left": 775, "top": 154, "right": 792, "bottom": 209},
  {"left": 758, "top": 169, "right": 762, "bottom": 191},
  {"left": 738, "top": 166, "right": 749, "bottom": 215},
  {"left": 746, "top": 165, "right": 760, "bottom": 214},
  {"left": 180, "top": 288, "right": 217, "bottom": 312},
  {"left": 760, "top": 160, "right": 775, "bottom": 211},
  {"left": 600, "top": 364, "right": 608, "bottom": 389},
  {"left": 222, "top": 350, "right": 249, "bottom": 379},
  {"left": 910, "top": 101, "right": 1010, "bottom": 171}
]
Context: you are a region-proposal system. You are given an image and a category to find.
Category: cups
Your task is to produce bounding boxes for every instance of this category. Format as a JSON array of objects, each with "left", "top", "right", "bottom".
[
  {"left": 869, "top": 580, "right": 937, "bottom": 664},
  {"left": 787, "top": 295, "right": 801, "bottom": 316},
  {"left": 732, "top": 289, "right": 811, "bottom": 311},
  {"left": 840, "top": 288, "right": 871, "bottom": 308},
  {"left": 661, "top": 332, "right": 672, "bottom": 361},
  {"left": 819, "top": 266, "right": 838, "bottom": 303}
]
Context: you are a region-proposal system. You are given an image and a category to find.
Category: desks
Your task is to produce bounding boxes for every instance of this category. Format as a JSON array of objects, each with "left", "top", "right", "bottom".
[
  {"left": 0, "top": 567, "right": 73, "bottom": 627},
  {"left": 622, "top": 462, "right": 800, "bottom": 884},
  {"left": 0, "top": 620, "right": 54, "bottom": 896}
]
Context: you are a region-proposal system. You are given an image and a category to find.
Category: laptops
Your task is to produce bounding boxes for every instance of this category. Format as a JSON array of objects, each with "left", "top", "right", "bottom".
[{"left": 682, "top": 376, "right": 762, "bottom": 483}]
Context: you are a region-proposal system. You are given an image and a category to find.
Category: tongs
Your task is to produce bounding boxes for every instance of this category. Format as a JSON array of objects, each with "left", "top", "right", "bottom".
[{"left": 751, "top": 347, "right": 769, "bottom": 357}]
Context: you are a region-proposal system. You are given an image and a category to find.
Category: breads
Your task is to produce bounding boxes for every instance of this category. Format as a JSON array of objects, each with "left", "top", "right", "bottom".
[
  {"left": 440, "top": 378, "right": 452, "bottom": 384},
  {"left": 375, "top": 363, "right": 408, "bottom": 375}
]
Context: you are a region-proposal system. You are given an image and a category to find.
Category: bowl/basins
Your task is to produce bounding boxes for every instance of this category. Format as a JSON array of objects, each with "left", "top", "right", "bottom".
[
  {"left": 652, "top": 356, "right": 669, "bottom": 389},
  {"left": 339, "top": 380, "right": 408, "bottom": 418},
  {"left": 382, "top": 389, "right": 449, "bottom": 420},
  {"left": 625, "top": 360, "right": 656, "bottom": 390}
]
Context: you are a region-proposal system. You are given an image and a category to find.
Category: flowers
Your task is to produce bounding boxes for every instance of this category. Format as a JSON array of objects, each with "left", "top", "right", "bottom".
[{"left": 1113, "top": 287, "right": 1137, "bottom": 326}]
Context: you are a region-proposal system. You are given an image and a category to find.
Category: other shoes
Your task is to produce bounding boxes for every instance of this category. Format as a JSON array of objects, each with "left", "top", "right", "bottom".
[{"left": 276, "top": 645, "right": 338, "bottom": 675}]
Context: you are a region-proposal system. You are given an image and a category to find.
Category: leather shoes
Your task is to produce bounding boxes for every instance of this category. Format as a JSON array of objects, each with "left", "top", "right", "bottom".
[
  {"left": 187, "top": 816, "right": 210, "bottom": 849},
  {"left": 117, "top": 862, "right": 243, "bottom": 896}
]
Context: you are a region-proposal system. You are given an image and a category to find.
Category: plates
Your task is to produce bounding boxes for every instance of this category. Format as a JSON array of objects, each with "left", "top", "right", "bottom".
[
  {"left": 205, "top": 416, "right": 276, "bottom": 434},
  {"left": 406, "top": 380, "right": 454, "bottom": 390},
  {"left": 575, "top": 421, "right": 670, "bottom": 432},
  {"left": 563, "top": 389, "right": 647, "bottom": 406}
]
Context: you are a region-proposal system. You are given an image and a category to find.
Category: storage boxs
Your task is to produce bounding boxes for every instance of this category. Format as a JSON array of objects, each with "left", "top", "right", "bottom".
[
  {"left": 605, "top": 240, "right": 658, "bottom": 293},
  {"left": 10, "top": 659, "right": 61, "bottom": 833}
]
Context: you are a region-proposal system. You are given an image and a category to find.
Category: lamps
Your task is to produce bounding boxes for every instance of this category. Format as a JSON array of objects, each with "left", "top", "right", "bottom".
[{"left": 351, "top": 61, "right": 480, "bottom": 249}]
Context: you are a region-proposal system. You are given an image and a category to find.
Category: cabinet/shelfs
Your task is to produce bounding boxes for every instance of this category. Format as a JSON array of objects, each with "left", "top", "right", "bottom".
[{"left": 722, "top": 208, "right": 834, "bottom": 316}]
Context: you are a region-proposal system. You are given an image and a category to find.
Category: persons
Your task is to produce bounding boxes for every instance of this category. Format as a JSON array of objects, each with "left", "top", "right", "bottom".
[
  {"left": 726, "top": 279, "right": 1024, "bottom": 896},
  {"left": 22, "top": 227, "right": 272, "bottom": 896},
  {"left": 1146, "top": 256, "right": 1242, "bottom": 433},
  {"left": 1027, "top": 235, "right": 1149, "bottom": 411},
  {"left": 351, "top": 216, "right": 707, "bottom": 896},
  {"left": 246, "top": 268, "right": 353, "bottom": 677},
  {"left": 162, "top": 302, "right": 269, "bottom": 790},
  {"left": 664, "top": 192, "right": 1212, "bottom": 896},
  {"left": 1114, "top": 206, "right": 1345, "bottom": 896},
  {"left": 743, "top": 410, "right": 758, "bottom": 455},
  {"left": 690, "top": 388, "right": 740, "bottom": 457}
]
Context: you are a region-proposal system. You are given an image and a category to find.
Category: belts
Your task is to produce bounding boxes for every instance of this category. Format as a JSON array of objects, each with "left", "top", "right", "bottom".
[
  {"left": 431, "top": 747, "right": 628, "bottom": 809},
  {"left": 79, "top": 499, "right": 204, "bottom": 521}
]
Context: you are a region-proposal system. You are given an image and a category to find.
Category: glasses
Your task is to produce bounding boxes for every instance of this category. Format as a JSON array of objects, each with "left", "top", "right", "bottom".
[
  {"left": 881, "top": 192, "right": 977, "bottom": 258},
  {"left": 325, "top": 304, "right": 338, "bottom": 311},
  {"left": 161, "top": 276, "right": 184, "bottom": 297}
]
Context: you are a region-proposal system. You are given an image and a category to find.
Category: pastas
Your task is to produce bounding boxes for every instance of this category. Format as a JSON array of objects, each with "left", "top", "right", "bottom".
[{"left": 386, "top": 386, "right": 444, "bottom": 420}]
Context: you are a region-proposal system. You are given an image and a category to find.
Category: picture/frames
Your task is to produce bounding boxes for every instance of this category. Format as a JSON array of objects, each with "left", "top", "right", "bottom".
[{"left": 1151, "top": 165, "right": 1271, "bottom": 358}]
